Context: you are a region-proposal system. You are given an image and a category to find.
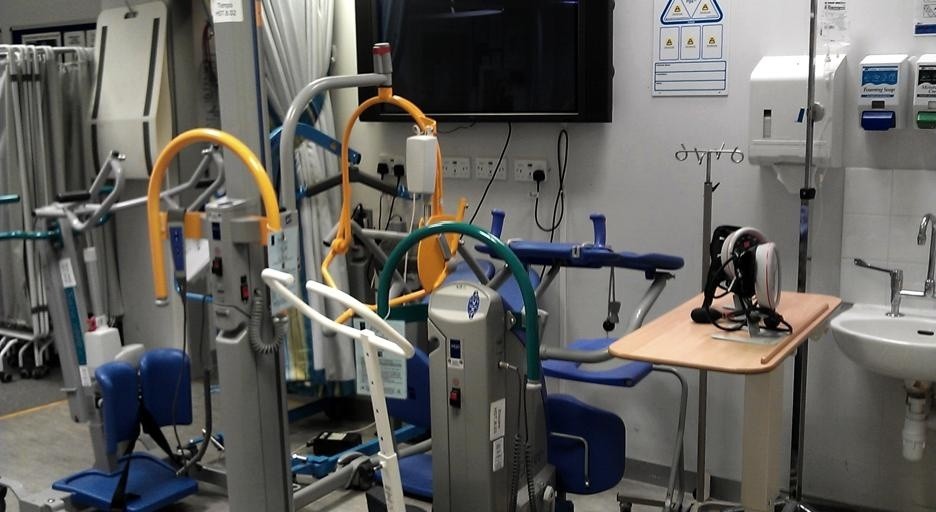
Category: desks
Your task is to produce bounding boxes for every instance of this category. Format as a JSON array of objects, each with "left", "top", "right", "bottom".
[{"left": 607, "top": 286, "right": 845, "bottom": 510}]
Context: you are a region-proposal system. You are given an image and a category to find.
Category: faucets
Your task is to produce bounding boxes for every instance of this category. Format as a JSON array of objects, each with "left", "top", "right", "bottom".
[{"left": 916, "top": 211, "right": 936, "bottom": 299}]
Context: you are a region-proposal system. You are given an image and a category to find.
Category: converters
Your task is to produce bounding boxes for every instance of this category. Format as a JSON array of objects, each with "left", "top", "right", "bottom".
[{"left": 311, "top": 431, "right": 362, "bottom": 457}]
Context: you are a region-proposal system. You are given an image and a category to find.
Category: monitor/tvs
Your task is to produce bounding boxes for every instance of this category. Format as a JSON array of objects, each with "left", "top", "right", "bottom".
[{"left": 354, "top": 0, "right": 615, "bottom": 123}]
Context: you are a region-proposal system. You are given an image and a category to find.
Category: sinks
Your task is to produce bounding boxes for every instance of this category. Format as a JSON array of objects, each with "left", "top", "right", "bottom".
[{"left": 829, "top": 301, "right": 936, "bottom": 380}]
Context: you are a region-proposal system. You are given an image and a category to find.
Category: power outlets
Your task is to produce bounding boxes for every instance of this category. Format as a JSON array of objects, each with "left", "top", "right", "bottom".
[
  {"left": 442, "top": 156, "right": 547, "bottom": 183},
  {"left": 376, "top": 154, "right": 406, "bottom": 177}
]
